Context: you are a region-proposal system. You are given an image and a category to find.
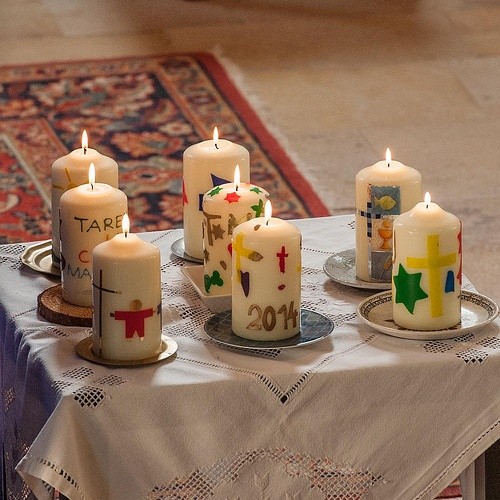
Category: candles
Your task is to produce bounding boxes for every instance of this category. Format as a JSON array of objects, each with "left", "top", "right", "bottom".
[
  {"left": 392, "top": 192, "right": 463, "bottom": 329},
  {"left": 59, "top": 162, "right": 127, "bottom": 308},
  {"left": 182, "top": 126, "right": 250, "bottom": 259},
  {"left": 231, "top": 201, "right": 305, "bottom": 341},
  {"left": 201, "top": 164, "right": 272, "bottom": 296},
  {"left": 51, "top": 130, "right": 119, "bottom": 269},
  {"left": 93, "top": 214, "right": 163, "bottom": 361},
  {"left": 354, "top": 147, "right": 422, "bottom": 282}
]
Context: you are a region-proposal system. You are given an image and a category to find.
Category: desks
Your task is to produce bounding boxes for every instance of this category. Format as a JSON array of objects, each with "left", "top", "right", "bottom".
[{"left": 0, "top": 214, "right": 500, "bottom": 500}]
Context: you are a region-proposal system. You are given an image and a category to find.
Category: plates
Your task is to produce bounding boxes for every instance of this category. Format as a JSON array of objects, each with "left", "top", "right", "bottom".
[
  {"left": 322, "top": 248, "right": 392, "bottom": 290},
  {"left": 202, "top": 308, "right": 335, "bottom": 350},
  {"left": 180, "top": 264, "right": 232, "bottom": 314},
  {"left": 74, "top": 335, "right": 179, "bottom": 366},
  {"left": 170, "top": 237, "right": 206, "bottom": 263},
  {"left": 20, "top": 237, "right": 61, "bottom": 277},
  {"left": 356, "top": 290, "right": 500, "bottom": 340}
]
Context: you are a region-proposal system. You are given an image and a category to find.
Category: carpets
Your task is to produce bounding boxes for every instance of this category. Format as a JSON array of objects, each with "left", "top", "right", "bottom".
[{"left": 0, "top": 50, "right": 330, "bottom": 244}]
